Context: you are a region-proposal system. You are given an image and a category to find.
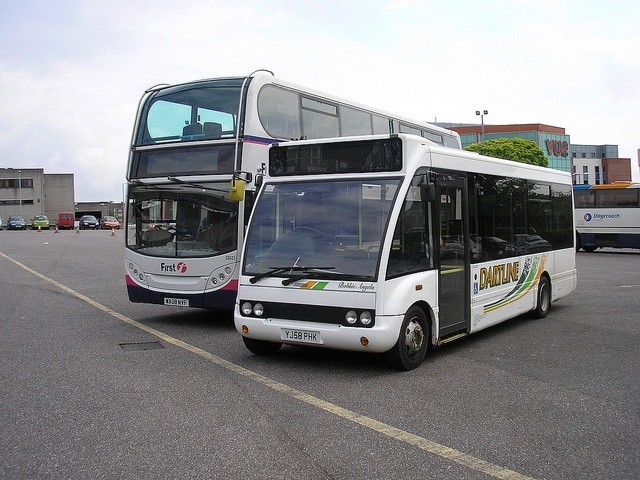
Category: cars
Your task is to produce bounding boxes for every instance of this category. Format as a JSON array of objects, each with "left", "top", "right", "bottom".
[
  {"left": 31, "top": 215, "right": 50, "bottom": 230},
  {"left": 7, "top": 216, "right": 26, "bottom": 230},
  {"left": 79, "top": 215, "right": 99, "bottom": 230},
  {"left": 101, "top": 216, "right": 120, "bottom": 230}
]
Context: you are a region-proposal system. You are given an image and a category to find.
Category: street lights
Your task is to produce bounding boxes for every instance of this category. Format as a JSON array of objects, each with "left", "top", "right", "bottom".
[{"left": 476, "top": 110, "right": 488, "bottom": 143}]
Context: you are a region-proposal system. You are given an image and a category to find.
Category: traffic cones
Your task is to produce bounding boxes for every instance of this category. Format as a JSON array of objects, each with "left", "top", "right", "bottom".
[
  {"left": 37, "top": 225, "right": 42, "bottom": 232},
  {"left": 111, "top": 228, "right": 115, "bottom": 236},
  {"left": 55, "top": 225, "right": 59, "bottom": 233},
  {"left": 77, "top": 226, "right": 80, "bottom": 234}
]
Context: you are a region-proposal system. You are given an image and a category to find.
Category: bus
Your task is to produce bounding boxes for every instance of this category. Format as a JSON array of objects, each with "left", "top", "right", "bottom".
[
  {"left": 235, "top": 136, "right": 577, "bottom": 373},
  {"left": 123, "top": 69, "right": 462, "bottom": 313},
  {"left": 570, "top": 180, "right": 639, "bottom": 253}
]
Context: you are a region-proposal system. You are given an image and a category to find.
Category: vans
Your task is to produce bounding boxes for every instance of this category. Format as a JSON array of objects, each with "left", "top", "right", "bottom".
[{"left": 57, "top": 212, "right": 74, "bottom": 230}]
